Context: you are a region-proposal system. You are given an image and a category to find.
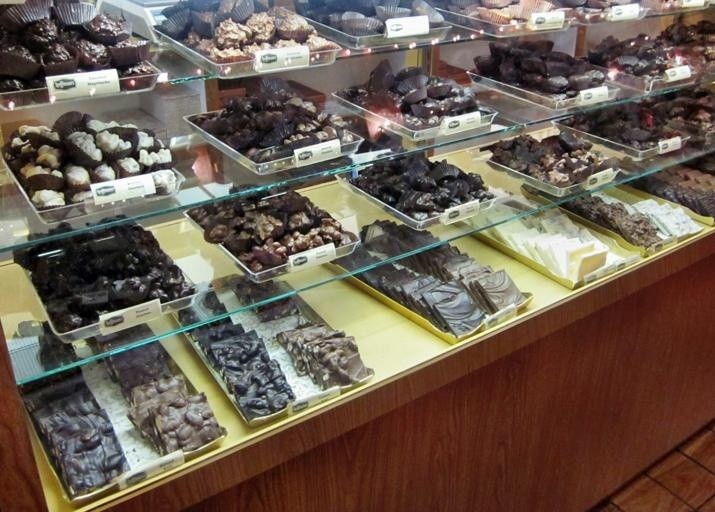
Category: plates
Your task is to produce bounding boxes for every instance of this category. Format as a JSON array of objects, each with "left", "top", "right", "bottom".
[
  {"left": 437, "top": 7, "right": 569, "bottom": 38},
  {"left": 561, "top": 6, "right": 651, "bottom": 28},
  {"left": 468, "top": 64, "right": 617, "bottom": 112},
  {"left": 184, "top": 107, "right": 365, "bottom": 173},
  {"left": 300, "top": 7, "right": 455, "bottom": 48},
  {"left": 517, "top": 182, "right": 705, "bottom": 257},
  {"left": 486, "top": 143, "right": 619, "bottom": 195},
  {"left": 168, "top": 281, "right": 375, "bottom": 426},
  {"left": 331, "top": 81, "right": 497, "bottom": 141},
  {"left": 461, "top": 199, "right": 627, "bottom": 291},
  {"left": 145, "top": 6, "right": 343, "bottom": 79},
  {"left": 324, "top": 258, "right": 534, "bottom": 348},
  {"left": 0, "top": 315, "right": 229, "bottom": 506},
  {"left": 0, "top": 133, "right": 182, "bottom": 227},
  {"left": 615, "top": 178, "right": 715, "bottom": 227},
  {"left": 184, "top": 192, "right": 359, "bottom": 283},
  {"left": 12, "top": 216, "right": 197, "bottom": 340},
  {"left": 337, "top": 158, "right": 513, "bottom": 233},
  {"left": 585, "top": 52, "right": 702, "bottom": 95},
  {"left": 0, "top": 2, "right": 161, "bottom": 112},
  {"left": 550, "top": 115, "right": 687, "bottom": 160}
]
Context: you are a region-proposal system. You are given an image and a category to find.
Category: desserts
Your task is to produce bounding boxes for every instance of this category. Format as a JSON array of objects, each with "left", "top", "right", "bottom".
[
  {"left": 152, "top": 0, "right": 342, "bottom": 80},
  {"left": 586, "top": 19, "right": 714, "bottom": 91},
  {"left": 473, "top": 39, "right": 605, "bottom": 108},
  {"left": 189, "top": 185, "right": 343, "bottom": 277},
  {"left": 335, "top": 58, "right": 499, "bottom": 140},
  {"left": 295, "top": 0, "right": 453, "bottom": 49},
  {"left": 2, "top": 1, "right": 160, "bottom": 101},
  {"left": 348, "top": 148, "right": 495, "bottom": 230},
  {"left": 2, "top": 111, "right": 186, "bottom": 224},
  {"left": 480, "top": 132, "right": 622, "bottom": 194},
  {"left": 571, "top": 88, "right": 714, "bottom": 159},
  {"left": 12, "top": 213, "right": 198, "bottom": 344},
  {"left": 432, "top": 1, "right": 710, "bottom": 32},
  {"left": 184, "top": 90, "right": 354, "bottom": 174}
]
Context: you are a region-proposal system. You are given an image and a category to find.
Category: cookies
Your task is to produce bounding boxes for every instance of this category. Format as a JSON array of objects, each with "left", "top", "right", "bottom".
[
  {"left": 327, "top": 220, "right": 528, "bottom": 340},
  {"left": 482, "top": 203, "right": 610, "bottom": 284},
  {"left": 532, "top": 180, "right": 705, "bottom": 249},
  {"left": 627, "top": 164, "right": 714, "bottom": 221},
  {"left": 171, "top": 279, "right": 373, "bottom": 424},
  {"left": 17, "top": 322, "right": 222, "bottom": 497}
]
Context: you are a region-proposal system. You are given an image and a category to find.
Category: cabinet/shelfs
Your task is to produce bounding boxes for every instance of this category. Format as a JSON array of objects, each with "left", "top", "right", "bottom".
[{"left": 0, "top": 0, "right": 715, "bottom": 511}]
